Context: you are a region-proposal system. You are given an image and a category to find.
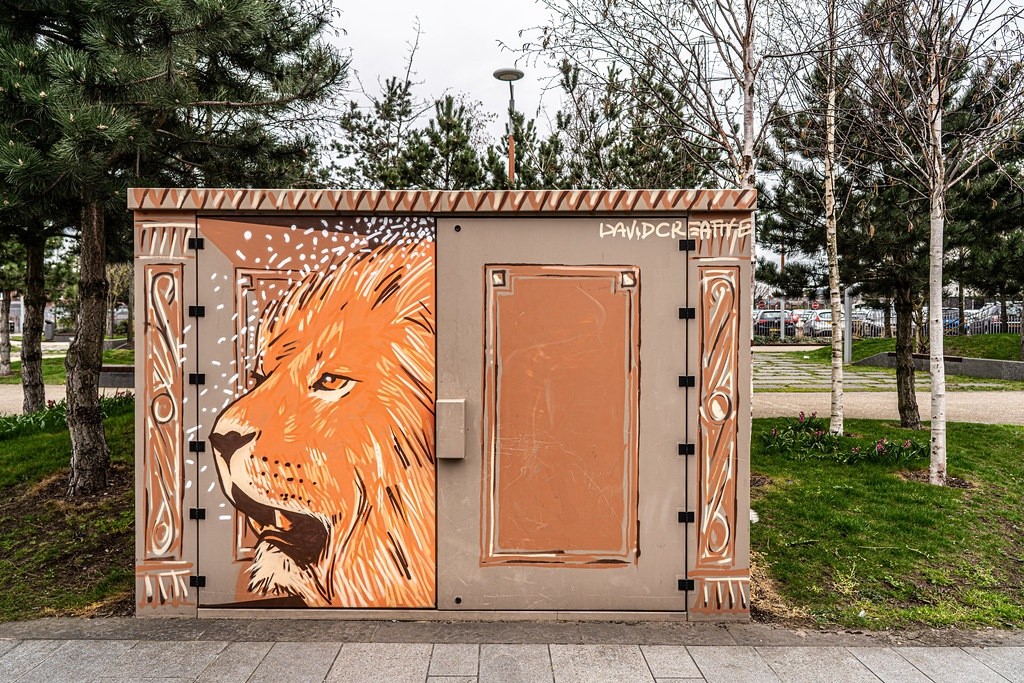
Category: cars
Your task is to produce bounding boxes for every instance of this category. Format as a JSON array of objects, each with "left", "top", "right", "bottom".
[{"left": 752, "top": 301, "right": 1024, "bottom": 338}]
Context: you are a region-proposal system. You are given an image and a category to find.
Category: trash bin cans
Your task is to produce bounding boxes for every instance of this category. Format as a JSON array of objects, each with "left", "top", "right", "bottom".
[{"left": 45, "top": 319, "right": 55, "bottom": 340}]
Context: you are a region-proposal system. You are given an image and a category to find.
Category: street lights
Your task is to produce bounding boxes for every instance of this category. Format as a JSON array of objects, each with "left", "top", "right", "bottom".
[{"left": 492, "top": 67, "right": 524, "bottom": 187}]
